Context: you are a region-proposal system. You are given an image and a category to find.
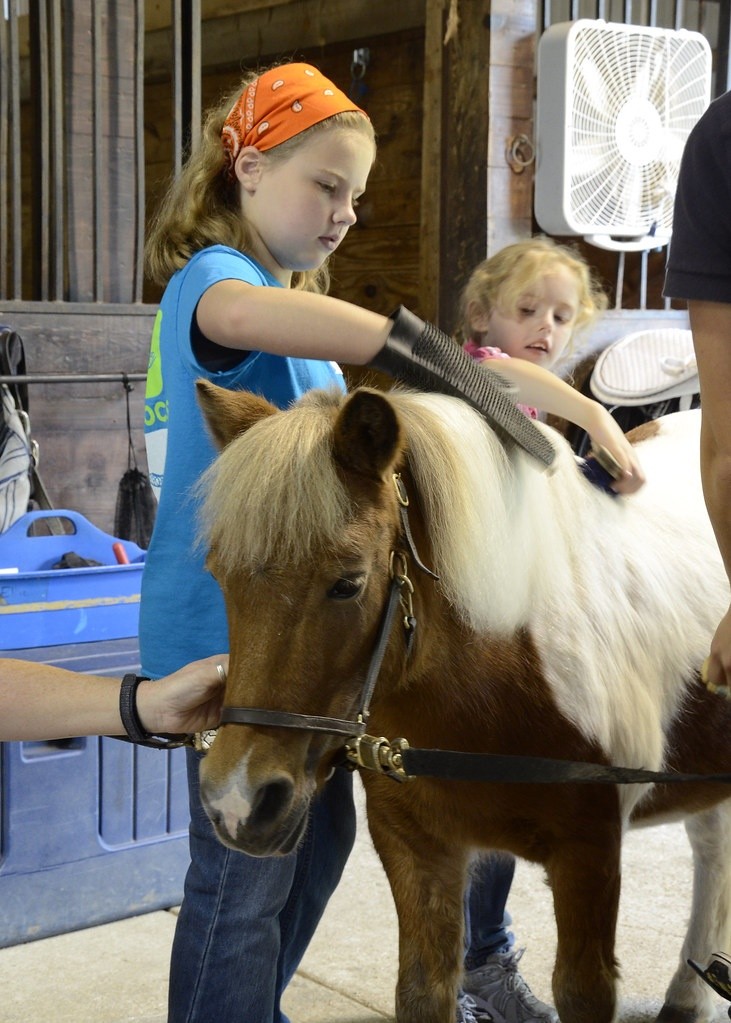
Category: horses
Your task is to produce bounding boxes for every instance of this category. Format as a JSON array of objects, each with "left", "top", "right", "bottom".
[{"left": 194, "top": 375, "right": 731, "bottom": 1022}]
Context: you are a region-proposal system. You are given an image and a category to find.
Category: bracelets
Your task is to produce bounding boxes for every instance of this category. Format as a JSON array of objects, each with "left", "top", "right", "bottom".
[{"left": 119, "top": 673, "right": 154, "bottom": 742}]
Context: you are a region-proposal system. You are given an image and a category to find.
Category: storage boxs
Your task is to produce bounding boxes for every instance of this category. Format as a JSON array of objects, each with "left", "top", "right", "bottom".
[
  {"left": 0, "top": 637, "right": 191, "bottom": 949},
  {"left": 0, "top": 509, "right": 147, "bottom": 651}
]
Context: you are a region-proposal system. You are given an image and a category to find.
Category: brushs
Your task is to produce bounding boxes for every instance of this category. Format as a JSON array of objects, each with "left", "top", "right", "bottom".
[{"left": 578, "top": 440, "right": 623, "bottom": 499}]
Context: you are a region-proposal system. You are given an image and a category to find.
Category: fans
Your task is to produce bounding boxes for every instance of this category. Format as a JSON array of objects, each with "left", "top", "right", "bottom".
[{"left": 533, "top": 18, "right": 712, "bottom": 253}]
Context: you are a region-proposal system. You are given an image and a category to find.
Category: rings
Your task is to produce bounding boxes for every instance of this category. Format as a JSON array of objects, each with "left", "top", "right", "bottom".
[{"left": 216, "top": 664, "right": 227, "bottom": 682}]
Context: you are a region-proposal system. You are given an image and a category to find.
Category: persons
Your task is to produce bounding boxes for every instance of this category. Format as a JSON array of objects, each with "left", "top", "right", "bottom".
[
  {"left": 140, "top": 63, "right": 554, "bottom": 1023},
  {"left": 454, "top": 234, "right": 646, "bottom": 1023},
  {"left": 1, "top": 652, "right": 231, "bottom": 742},
  {"left": 661, "top": 89, "right": 731, "bottom": 688}
]
